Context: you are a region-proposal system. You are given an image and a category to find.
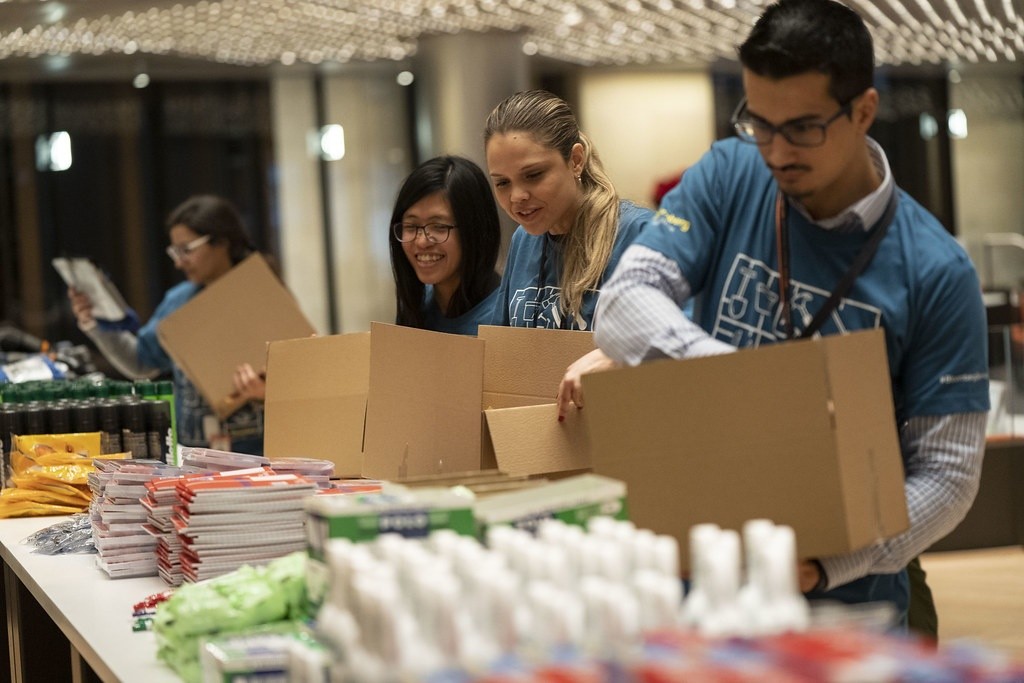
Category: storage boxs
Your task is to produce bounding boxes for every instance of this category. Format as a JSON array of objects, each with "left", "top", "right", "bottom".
[
  {"left": 263, "top": 321, "right": 486, "bottom": 482},
  {"left": 474, "top": 323, "right": 591, "bottom": 480},
  {"left": 305, "top": 504, "right": 479, "bottom": 561},
  {"left": 200, "top": 622, "right": 325, "bottom": 683},
  {"left": 153, "top": 252, "right": 317, "bottom": 420},
  {"left": 477, "top": 473, "right": 627, "bottom": 545},
  {"left": 580, "top": 328, "right": 911, "bottom": 576}
]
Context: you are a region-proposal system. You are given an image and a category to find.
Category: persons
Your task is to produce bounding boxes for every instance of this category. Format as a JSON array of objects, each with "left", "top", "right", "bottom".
[
  {"left": 482, "top": 89, "right": 695, "bottom": 331},
  {"left": 387, "top": 157, "right": 504, "bottom": 337},
  {"left": 594, "top": 1, "right": 991, "bottom": 639},
  {"left": 65, "top": 196, "right": 269, "bottom": 457}
]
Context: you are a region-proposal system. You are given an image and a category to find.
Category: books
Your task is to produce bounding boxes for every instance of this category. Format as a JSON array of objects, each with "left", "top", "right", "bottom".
[{"left": 87, "top": 445, "right": 383, "bottom": 587}]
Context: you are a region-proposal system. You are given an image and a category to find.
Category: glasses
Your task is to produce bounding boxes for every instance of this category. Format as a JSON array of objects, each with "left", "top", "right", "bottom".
[
  {"left": 390, "top": 219, "right": 458, "bottom": 246},
  {"left": 166, "top": 233, "right": 215, "bottom": 263},
  {"left": 731, "top": 93, "right": 855, "bottom": 147}
]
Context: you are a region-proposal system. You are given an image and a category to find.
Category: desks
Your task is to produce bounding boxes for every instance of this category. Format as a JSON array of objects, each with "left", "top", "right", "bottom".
[{"left": 0, "top": 443, "right": 182, "bottom": 683}]
